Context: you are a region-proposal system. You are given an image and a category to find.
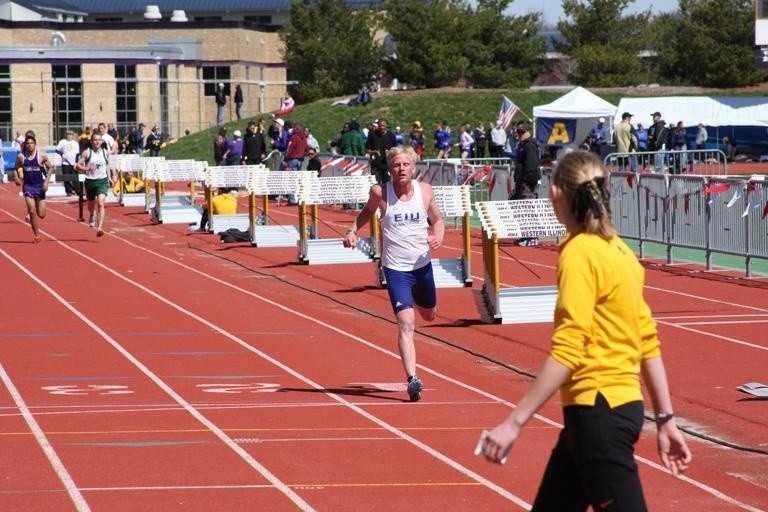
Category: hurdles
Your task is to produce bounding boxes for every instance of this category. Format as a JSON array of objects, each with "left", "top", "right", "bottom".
[
  {"left": 102, "top": 153, "right": 269, "bottom": 236},
  {"left": 42, "top": 151, "right": 86, "bottom": 221},
  {"left": 379, "top": 184, "right": 475, "bottom": 288},
  {"left": 246, "top": 171, "right": 378, "bottom": 266},
  {"left": 475, "top": 197, "right": 570, "bottom": 323}
]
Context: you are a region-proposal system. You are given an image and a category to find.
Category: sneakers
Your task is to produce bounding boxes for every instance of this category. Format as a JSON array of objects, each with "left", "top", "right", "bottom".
[
  {"left": 97, "top": 228, "right": 104, "bottom": 237},
  {"left": 408, "top": 377, "right": 422, "bottom": 401},
  {"left": 89, "top": 215, "right": 95, "bottom": 227}
]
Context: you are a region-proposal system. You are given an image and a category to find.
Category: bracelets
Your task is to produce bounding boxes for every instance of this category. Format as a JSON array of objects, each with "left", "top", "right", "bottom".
[{"left": 655, "top": 413, "right": 673, "bottom": 425}]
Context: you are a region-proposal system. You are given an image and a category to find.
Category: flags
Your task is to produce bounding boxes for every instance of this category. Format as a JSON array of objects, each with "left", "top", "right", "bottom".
[{"left": 497, "top": 97, "right": 519, "bottom": 131}]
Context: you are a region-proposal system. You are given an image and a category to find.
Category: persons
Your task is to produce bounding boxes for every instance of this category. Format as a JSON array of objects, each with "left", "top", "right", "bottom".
[
  {"left": 355, "top": 82, "right": 370, "bottom": 105},
  {"left": 481, "top": 151, "right": 693, "bottom": 512},
  {"left": 11, "top": 122, "right": 165, "bottom": 243},
  {"left": 341, "top": 144, "right": 445, "bottom": 403},
  {"left": 268, "top": 93, "right": 296, "bottom": 119},
  {"left": 215, "top": 82, "right": 227, "bottom": 128},
  {"left": 233, "top": 82, "right": 245, "bottom": 122},
  {"left": 330, "top": 96, "right": 356, "bottom": 107}
]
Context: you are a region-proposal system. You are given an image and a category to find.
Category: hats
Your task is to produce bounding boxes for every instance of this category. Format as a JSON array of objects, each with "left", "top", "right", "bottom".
[
  {"left": 518, "top": 124, "right": 529, "bottom": 132},
  {"left": 650, "top": 112, "right": 661, "bottom": 116},
  {"left": 233, "top": 130, "right": 242, "bottom": 137},
  {"left": 622, "top": 113, "right": 632, "bottom": 118},
  {"left": 274, "top": 118, "right": 284, "bottom": 127}
]
